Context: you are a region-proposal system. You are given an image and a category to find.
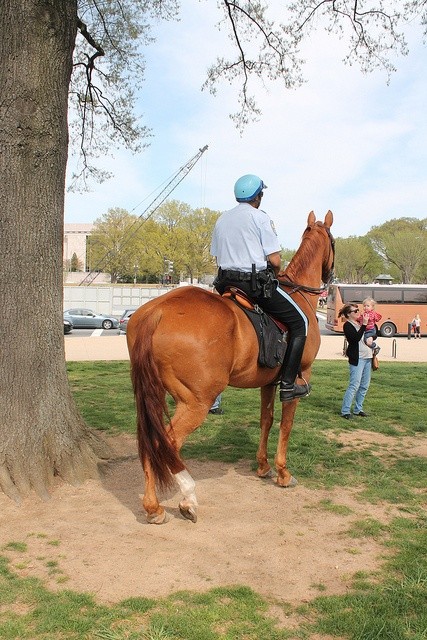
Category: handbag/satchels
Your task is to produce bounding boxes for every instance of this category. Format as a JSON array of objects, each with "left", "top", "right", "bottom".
[{"left": 373, "top": 352, "right": 379, "bottom": 371}]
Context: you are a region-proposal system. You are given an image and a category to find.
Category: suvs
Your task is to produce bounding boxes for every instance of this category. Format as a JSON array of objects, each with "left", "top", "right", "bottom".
[{"left": 120, "top": 309, "right": 136, "bottom": 332}]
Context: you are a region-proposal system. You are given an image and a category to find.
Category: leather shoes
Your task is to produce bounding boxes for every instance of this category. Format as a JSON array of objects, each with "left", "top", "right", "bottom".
[{"left": 279, "top": 385, "right": 312, "bottom": 401}]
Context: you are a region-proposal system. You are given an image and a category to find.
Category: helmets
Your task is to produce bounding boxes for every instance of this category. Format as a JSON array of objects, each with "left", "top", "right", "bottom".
[{"left": 234, "top": 174, "right": 267, "bottom": 202}]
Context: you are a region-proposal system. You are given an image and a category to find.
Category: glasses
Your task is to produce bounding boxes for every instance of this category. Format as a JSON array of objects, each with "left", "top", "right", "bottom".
[{"left": 349, "top": 308, "right": 359, "bottom": 313}]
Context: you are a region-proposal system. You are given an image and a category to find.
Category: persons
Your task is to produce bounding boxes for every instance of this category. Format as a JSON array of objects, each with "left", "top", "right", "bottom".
[
  {"left": 338, "top": 304, "right": 374, "bottom": 418},
  {"left": 359, "top": 298, "right": 381, "bottom": 356},
  {"left": 209, "top": 391, "right": 223, "bottom": 414},
  {"left": 210, "top": 174, "right": 312, "bottom": 402},
  {"left": 319, "top": 288, "right": 328, "bottom": 305},
  {"left": 411, "top": 314, "right": 421, "bottom": 339}
]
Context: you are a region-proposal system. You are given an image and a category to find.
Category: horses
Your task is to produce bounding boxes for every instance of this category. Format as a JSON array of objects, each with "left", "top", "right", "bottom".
[{"left": 125, "top": 209, "right": 337, "bottom": 526}]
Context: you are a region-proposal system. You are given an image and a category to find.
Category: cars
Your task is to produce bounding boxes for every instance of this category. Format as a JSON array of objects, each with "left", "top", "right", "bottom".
[
  {"left": 63, "top": 314, "right": 74, "bottom": 334},
  {"left": 63, "top": 307, "right": 119, "bottom": 330}
]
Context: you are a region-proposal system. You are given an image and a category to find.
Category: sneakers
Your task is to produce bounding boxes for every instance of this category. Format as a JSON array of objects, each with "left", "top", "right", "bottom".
[
  {"left": 344, "top": 414, "right": 355, "bottom": 420},
  {"left": 208, "top": 408, "right": 225, "bottom": 415},
  {"left": 359, "top": 412, "right": 367, "bottom": 416},
  {"left": 374, "top": 345, "right": 380, "bottom": 356}
]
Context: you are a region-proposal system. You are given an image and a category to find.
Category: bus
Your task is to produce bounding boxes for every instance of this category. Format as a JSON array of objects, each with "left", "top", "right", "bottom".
[{"left": 324, "top": 283, "right": 427, "bottom": 337}]
